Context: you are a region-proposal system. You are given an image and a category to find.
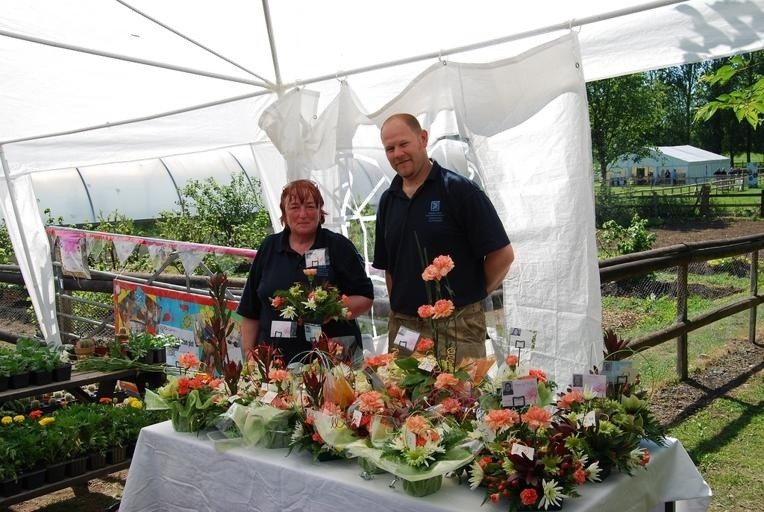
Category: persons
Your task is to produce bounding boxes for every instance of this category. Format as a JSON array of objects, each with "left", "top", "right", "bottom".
[
  {"left": 369, "top": 113, "right": 514, "bottom": 366},
  {"left": 634, "top": 166, "right": 742, "bottom": 186},
  {"left": 235, "top": 180, "right": 375, "bottom": 373}
]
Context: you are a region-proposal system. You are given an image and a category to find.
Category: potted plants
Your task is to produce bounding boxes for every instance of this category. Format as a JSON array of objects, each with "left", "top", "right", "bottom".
[{"left": 2, "top": 340, "right": 169, "bottom": 499}]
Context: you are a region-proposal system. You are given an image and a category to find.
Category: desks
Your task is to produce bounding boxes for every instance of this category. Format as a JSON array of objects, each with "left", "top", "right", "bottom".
[{"left": 120, "top": 419, "right": 714, "bottom": 512}]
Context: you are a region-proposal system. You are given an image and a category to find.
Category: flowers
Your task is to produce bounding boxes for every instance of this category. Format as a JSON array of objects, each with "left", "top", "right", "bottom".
[
  {"left": 420, "top": 256, "right": 455, "bottom": 280},
  {"left": 417, "top": 299, "right": 454, "bottom": 320},
  {"left": 162, "top": 337, "right": 651, "bottom": 512},
  {"left": 271, "top": 268, "right": 351, "bottom": 320}
]
